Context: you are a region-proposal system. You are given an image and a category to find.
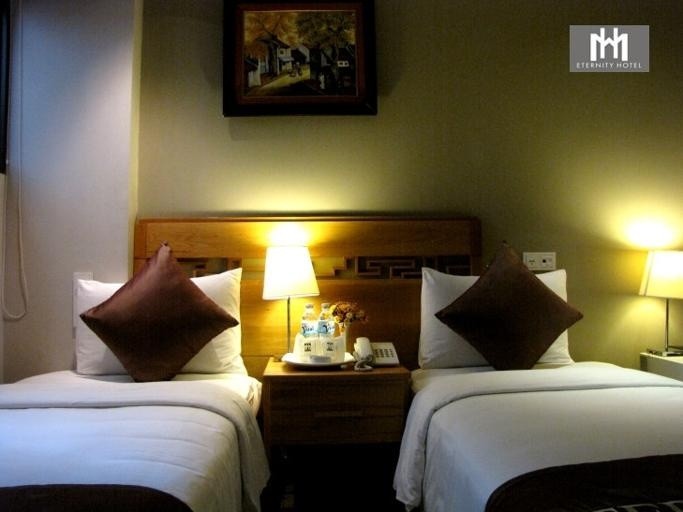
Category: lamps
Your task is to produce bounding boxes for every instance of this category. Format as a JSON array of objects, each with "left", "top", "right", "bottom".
[
  {"left": 262, "top": 246, "right": 320, "bottom": 360},
  {"left": 638, "top": 250, "right": 683, "bottom": 356}
]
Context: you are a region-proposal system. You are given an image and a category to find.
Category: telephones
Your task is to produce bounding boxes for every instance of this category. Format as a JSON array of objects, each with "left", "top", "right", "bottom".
[{"left": 352, "top": 337, "right": 399, "bottom": 372}]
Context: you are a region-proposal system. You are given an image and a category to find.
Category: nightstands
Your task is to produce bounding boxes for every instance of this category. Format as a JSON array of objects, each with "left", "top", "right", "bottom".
[{"left": 262, "top": 357, "right": 410, "bottom": 467}]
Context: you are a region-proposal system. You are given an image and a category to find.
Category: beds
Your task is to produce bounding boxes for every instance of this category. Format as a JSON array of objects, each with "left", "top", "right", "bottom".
[
  {"left": 393, "top": 361, "right": 683, "bottom": 512},
  {"left": 0, "top": 366, "right": 272, "bottom": 512}
]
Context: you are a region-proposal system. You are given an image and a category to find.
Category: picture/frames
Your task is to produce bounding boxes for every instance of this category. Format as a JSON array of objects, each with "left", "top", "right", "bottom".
[{"left": 223, "top": 0, "right": 378, "bottom": 116}]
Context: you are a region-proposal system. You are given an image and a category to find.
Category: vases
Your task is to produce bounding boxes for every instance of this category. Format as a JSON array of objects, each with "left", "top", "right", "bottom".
[{"left": 339, "top": 326, "right": 351, "bottom": 369}]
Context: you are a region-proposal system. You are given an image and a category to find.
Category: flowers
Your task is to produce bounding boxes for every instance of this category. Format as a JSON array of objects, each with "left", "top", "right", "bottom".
[{"left": 324, "top": 300, "right": 369, "bottom": 331}]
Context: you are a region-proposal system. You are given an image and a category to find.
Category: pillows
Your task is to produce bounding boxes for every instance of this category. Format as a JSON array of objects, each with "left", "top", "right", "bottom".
[
  {"left": 417, "top": 266, "right": 574, "bottom": 367},
  {"left": 434, "top": 241, "right": 583, "bottom": 369},
  {"left": 79, "top": 241, "right": 239, "bottom": 382},
  {"left": 77, "top": 267, "right": 249, "bottom": 375}
]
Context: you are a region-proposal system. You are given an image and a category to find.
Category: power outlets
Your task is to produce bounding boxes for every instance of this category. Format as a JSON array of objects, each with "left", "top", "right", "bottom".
[{"left": 523, "top": 252, "right": 555, "bottom": 271}]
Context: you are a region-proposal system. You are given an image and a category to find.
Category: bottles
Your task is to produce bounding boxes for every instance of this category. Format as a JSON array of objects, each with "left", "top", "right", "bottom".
[
  {"left": 317, "top": 302, "right": 335, "bottom": 362},
  {"left": 301, "top": 303, "right": 318, "bottom": 362}
]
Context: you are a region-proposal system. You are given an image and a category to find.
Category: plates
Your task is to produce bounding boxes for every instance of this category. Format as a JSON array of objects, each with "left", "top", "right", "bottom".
[{"left": 281, "top": 351, "right": 354, "bottom": 367}]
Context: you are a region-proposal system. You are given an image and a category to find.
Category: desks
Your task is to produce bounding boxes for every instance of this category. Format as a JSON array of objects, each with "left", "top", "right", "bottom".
[{"left": 640, "top": 353, "right": 683, "bottom": 382}]
